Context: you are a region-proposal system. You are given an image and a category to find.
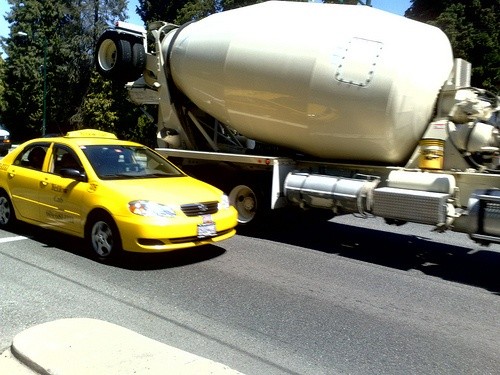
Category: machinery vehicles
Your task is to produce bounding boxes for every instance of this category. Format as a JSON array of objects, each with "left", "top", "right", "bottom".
[{"left": 94, "top": 0, "right": 500, "bottom": 254}]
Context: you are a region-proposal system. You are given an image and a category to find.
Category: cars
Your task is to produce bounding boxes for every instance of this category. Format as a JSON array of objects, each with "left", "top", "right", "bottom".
[{"left": 0, "top": 129, "right": 239, "bottom": 263}]
